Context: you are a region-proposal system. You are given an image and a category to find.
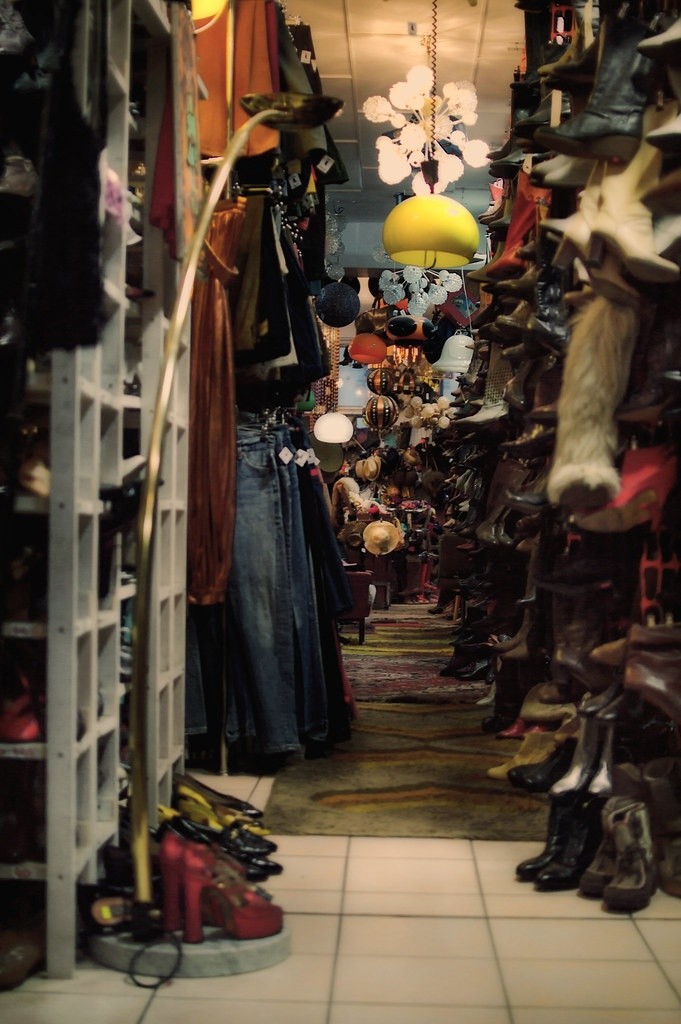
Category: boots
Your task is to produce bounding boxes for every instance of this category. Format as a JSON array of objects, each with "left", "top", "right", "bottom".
[
  {"left": 580, "top": 796, "right": 640, "bottom": 894},
  {"left": 445, "top": 0, "right": 680, "bottom": 754},
  {"left": 549, "top": 716, "right": 600, "bottom": 794},
  {"left": 587, "top": 719, "right": 617, "bottom": 795},
  {"left": 536, "top": 795, "right": 608, "bottom": 887},
  {"left": 642, "top": 755, "right": 680, "bottom": 898},
  {"left": 485, "top": 731, "right": 563, "bottom": 783},
  {"left": 604, "top": 801, "right": 659, "bottom": 913},
  {"left": 514, "top": 793, "right": 582, "bottom": 879}
]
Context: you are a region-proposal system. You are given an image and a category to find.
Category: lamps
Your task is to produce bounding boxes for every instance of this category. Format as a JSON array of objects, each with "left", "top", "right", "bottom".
[{"left": 287, "top": 0, "right": 490, "bottom": 446}]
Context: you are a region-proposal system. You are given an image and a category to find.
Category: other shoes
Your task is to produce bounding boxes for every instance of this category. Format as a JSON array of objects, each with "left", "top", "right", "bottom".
[
  {"left": 508, "top": 762, "right": 539, "bottom": 785},
  {"left": 523, "top": 736, "right": 577, "bottom": 790},
  {"left": 611, "top": 762, "right": 656, "bottom": 886}
]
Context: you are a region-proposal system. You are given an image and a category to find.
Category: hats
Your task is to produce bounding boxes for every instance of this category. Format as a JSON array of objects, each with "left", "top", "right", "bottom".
[
  {"left": 364, "top": 455, "right": 381, "bottom": 482},
  {"left": 338, "top": 521, "right": 368, "bottom": 551},
  {"left": 363, "top": 520, "right": 399, "bottom": 556},
  {"left": 356, "top": 459, "right": 368, "bottom": 482}
]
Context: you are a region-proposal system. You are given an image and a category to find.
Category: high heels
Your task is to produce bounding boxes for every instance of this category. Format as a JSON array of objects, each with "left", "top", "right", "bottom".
[
  {"left": 175, "top": 786, "right": 272, "bottom": 835},
  {"left": 161, "top": 832, "right": 283, "bottom": 944},
  {"left": 159, "top": 814, "right": 280, "bottom": 886},
  {"left": 177, "top": 772, "right": 263, "bottom": 818}
]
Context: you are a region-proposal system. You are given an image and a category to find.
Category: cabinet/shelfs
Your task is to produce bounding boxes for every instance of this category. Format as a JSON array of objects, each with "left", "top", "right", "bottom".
[{"left": 0, "top": 2, "right": 197, "bottom": 979}]
